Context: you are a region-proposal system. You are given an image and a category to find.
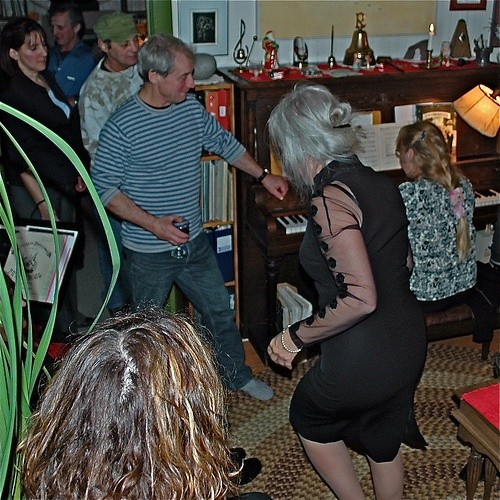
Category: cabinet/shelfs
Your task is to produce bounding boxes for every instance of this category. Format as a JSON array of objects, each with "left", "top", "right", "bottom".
[{"left": 185, "top": 72, "right": 240, "bottom": 331}]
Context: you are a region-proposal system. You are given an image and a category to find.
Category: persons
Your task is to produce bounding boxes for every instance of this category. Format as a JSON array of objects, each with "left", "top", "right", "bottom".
[
  {"left": 17, "top": 304, "right": 246, "bottom": 499},
  {"left": 395, "top": 118, "right": 478, "bottom": 302},
  {"left": 268, "top": 84, "right": 430, "bottom": 500},
  {"left": 91, "top": 33, "right": 287, "bottom": 401},
  {"left": 1, "top": 4, "right": 145, "bottom": 346}
]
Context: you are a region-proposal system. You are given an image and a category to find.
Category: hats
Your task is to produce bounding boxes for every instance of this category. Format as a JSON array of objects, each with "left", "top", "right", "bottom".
[{"left": 92, "top": 12, "right": 142, "bottom": 43}]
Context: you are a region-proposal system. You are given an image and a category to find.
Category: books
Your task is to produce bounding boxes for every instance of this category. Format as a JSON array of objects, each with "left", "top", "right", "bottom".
[{"left": 192, "top": 87, "right": 312, "bottom": 328}]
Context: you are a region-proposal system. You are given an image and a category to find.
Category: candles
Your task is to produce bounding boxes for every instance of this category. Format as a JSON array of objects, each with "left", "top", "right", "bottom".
[{"left": 427, "top": 23, "right": 434, "bottom": 51}]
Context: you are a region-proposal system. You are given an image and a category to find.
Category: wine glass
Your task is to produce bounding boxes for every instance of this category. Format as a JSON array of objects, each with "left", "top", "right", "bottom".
[{"left": 171, "top": 221, "right": 190, "bottom": 259}]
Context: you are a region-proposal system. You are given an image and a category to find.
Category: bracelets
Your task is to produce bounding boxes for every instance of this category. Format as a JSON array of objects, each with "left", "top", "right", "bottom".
[
  {"left": 257, "top": 169, "right": 267, "bottom": 181},
  {"left": 30, "top": 199, "right": 45, "bottom": 218},
  {"left": 282, "top": 328, "right": 304, "bottom": 352}
]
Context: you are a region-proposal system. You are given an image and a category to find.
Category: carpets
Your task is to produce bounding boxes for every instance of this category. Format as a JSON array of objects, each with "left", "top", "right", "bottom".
[{"left": 223, "top": 342, "right": 500, "bottom": 500}]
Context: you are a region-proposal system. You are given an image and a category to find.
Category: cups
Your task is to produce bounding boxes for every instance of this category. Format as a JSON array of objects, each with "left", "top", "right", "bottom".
[{"left": 475, "top": 48, "right": 491, "bottom": 67}]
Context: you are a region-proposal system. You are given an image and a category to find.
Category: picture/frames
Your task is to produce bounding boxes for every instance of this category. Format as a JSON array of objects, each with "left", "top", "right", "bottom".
[
  {"left": 177, "top": 0, "right": 229, "bottom": 57},
  {"left": 450, "top": 0, "right": 487, "bottom": 11}
]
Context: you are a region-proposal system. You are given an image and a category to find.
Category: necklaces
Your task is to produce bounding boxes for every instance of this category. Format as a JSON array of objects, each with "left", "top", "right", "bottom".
[{"left": 106, "top": 60, "right": 133, "bottom": 78}]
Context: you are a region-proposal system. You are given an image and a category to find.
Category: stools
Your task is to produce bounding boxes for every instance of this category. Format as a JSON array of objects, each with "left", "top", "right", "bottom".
[{"left": 422, "top": 285, "right": 496, "bottom": 361}]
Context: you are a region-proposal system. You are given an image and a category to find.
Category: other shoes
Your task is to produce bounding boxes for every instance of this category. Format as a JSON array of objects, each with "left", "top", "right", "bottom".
[
  {"left": 229, "top": 457, "right": 262, "bottom": 485},
  {"left": 226, "top": 447, "right": 246, "bottom": 460}
]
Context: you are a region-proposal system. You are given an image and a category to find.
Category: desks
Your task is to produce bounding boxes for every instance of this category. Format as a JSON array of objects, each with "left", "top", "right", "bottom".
[{"left": 450, "top": 377, "right": 500, "bottom": 500}]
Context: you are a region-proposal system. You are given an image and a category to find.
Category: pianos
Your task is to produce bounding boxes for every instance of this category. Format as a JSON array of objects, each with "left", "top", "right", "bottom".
[{"left": 216, "top": 59, "right": 500, "bottom": 380}]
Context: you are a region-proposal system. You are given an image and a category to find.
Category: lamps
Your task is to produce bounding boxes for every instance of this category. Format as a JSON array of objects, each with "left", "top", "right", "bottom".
[{"left": 453, "top": 84, "right": 500, "bottom": 137}]
